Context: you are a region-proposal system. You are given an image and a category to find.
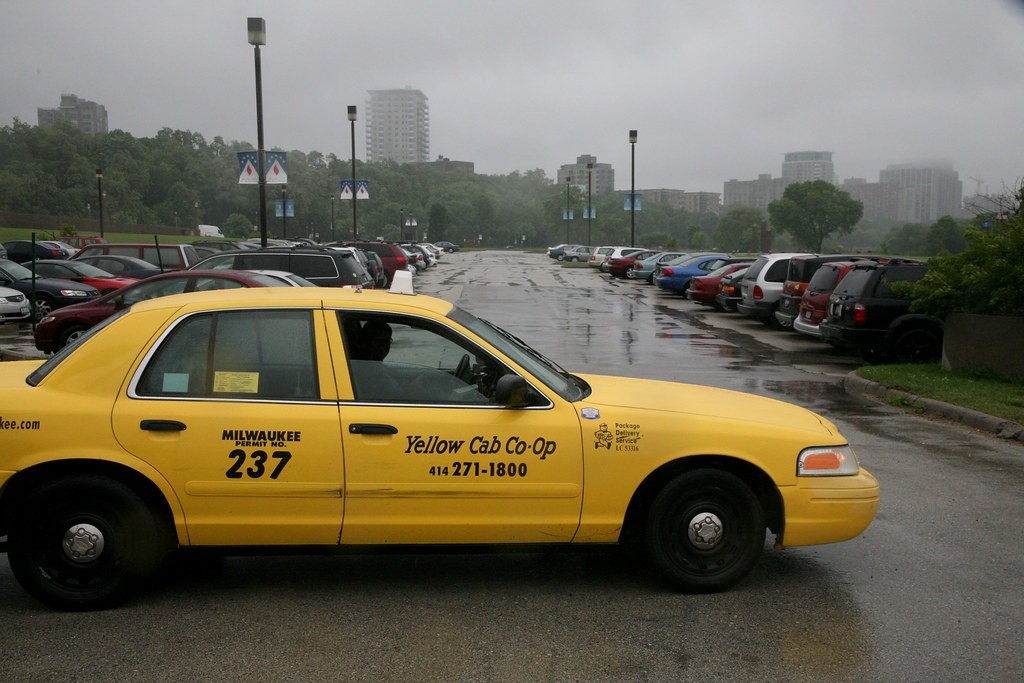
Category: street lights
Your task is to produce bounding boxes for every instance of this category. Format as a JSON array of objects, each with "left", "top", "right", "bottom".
[
  {"left": 248, "top": 17, "right": 266, "bottom": 246},
  {"left": 281, "top": 184, "right": 286, "bottom": 238},
  {"left": 400, "top": 209, "right": 412, "bottom": 241},
  {"left": 629, "top": 130, "right": 638, "bottom": 247},
  {"left": 566, "top": 176, "right": 571, "bottom": 243},
  {"left": 587, "top": 164, "right": 593, "bottom": 245},
  {"left": 96, "top": 170, "right": 104, "bottom": 238},
  {"left": 331, "top": 106, "right": 357, "bottom": 242}
]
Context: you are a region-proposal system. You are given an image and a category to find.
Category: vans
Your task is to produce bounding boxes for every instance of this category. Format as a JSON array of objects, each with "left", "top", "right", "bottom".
[
  {"left": 776, "top": 256, "right": 947, "bottom": 364},
  {"left": 68, "top": 243, "right": 199, "bottom": 271},
  {"left": 198, "top": 224, "right": 225, "bottom": 238}
]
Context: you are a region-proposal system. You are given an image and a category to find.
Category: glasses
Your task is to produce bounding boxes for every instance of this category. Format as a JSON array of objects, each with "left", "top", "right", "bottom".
[{"left": 366, "top": 336, "right": 393, "bottom": 345}]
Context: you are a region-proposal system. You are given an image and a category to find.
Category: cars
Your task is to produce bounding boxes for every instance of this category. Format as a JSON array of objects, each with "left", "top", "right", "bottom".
[
  {"left": 546, "top": 243, "right": 749, "bottom": 314},
  {"left": 395, "top": 241, "right": 461, "bottom": 275},
  {"left": 0, "top": 238, "right": 159, "bottom": 323},
  {"left": 192, "top": 238, "right": 316, "bottom": 262},
  {"left": 0, "top": 285, "right": 880, "bottom": 611},
  {"left": 35, "top": 270, "right": 319, "bottom": 355}
]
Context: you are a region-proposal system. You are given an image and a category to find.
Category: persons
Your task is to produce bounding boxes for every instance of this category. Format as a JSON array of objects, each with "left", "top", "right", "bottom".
[{"left": 354, "top": 320, "right": 434, "bottom": 400}]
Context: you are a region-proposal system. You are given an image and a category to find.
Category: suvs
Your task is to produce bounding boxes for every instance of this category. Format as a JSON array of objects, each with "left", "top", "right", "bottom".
[
  {"left": 736, "top": 251, "right": 823, "bottom": 327},
  {"left": 186, "top": 245, "right": 386, "bottom": 289},
  {"left": 327, "top": 239, "right": 409, "bottom": 288}
]
